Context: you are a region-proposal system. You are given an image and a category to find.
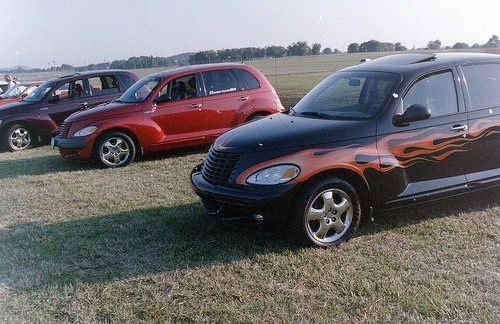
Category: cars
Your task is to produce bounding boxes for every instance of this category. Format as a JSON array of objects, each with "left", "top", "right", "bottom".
[
  {"left": 188, "top": 51, "right": 500, "bottom": 258},
  {"left": 0, "top": 81, "right": 38, "bottom": 96},
  {"left": 0, "top": 81, "right": 102, "bottom": 107},
  {"left": 30, "top": 82, "right": 69, "bottom": 94},
  {"left": 51, "top": 61, "right": 287, "bottom": 169},
  {"left": 0, "top": 69, "right": 159, "bottom": 152}
]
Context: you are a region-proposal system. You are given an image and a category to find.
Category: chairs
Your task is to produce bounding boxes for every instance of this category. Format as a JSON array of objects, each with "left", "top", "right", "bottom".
[
  {"left": 73, "top": 84, "right": 83, "bottom": 97},
  {"left": 172, "top": 81, "right": 187, "bottom": 100},
  {"left": 188, "top": 77, "right": 197, "bottom": 98}
]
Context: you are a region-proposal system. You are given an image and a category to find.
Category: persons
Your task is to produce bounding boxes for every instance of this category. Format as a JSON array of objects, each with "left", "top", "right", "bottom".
[{"left": 4, "top": 75, "right": 17, "bottom": 91}]
